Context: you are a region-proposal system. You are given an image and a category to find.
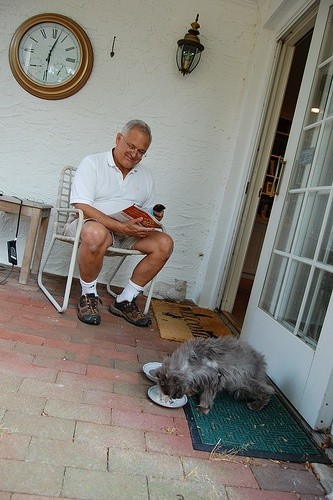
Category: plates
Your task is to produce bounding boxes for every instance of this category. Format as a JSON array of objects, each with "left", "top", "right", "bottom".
[
  {"left": 143, "top": 362, "right": 163, "bottom": 381},
  {"left": 148, "top": 386, "right": 187, "bottom": 408}
]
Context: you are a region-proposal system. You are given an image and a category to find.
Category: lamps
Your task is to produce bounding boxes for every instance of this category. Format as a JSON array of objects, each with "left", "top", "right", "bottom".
[{"left": 176, "top": 13, "right": 206, "bottom": 76}]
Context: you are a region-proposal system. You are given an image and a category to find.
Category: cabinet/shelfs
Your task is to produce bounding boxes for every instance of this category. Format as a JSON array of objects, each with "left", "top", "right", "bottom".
[{"left": 242, "top": 115, "right": 312, "bottom": 289}]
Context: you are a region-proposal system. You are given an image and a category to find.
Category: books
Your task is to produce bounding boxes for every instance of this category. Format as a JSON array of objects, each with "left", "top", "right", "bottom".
[{"left": 105, "top": 202, "right": 166, "bottom": 234}]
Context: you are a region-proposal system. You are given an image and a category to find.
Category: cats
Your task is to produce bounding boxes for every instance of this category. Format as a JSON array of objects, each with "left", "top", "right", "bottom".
[{"left": 156, "top": 335, "right": 274, "bottom": 414}]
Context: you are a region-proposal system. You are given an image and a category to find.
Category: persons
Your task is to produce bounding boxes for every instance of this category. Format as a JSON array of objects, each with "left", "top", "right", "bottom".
[{"left": 59, "top": 118, "right": 174, "bottom": 327}]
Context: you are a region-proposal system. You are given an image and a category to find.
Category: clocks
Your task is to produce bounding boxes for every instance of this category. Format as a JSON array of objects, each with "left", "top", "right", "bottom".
[{"left": 9, "top": 13, "right": 94, "bottom": 100}]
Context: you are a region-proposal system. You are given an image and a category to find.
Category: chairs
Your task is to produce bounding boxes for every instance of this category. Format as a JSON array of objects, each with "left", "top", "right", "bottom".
[{"left": 38, "top": 165, "right": 166, "bottom": 313}]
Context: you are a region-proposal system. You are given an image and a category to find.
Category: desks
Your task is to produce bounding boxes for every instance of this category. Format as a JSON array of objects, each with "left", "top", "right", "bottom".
[{"left": 0, "top": 194, "right": 54, "bottom": 284}]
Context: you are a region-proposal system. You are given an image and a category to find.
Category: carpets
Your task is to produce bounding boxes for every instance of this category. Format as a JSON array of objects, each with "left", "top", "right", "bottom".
[
  {"left": 150, "top": 299, "right": 232, "bottom": 345},
  {"left": 182, "top": 389, "right": 333, "bottom": 464}
]
